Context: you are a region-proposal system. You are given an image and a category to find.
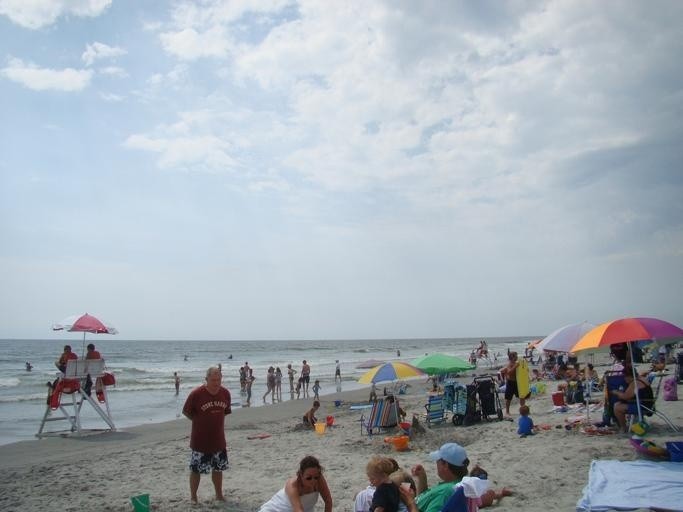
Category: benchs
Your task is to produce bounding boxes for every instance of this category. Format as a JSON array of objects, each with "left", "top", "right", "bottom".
[{"left": 54, "top": 358, "right": 105, "bottom": 380}]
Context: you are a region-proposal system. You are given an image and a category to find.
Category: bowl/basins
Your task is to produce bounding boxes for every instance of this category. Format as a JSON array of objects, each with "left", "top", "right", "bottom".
[{"left": 399, "top": 422, "right": 409, "bottom": 428}]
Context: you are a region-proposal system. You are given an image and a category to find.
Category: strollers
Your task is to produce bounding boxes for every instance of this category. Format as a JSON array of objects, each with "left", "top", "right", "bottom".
[
  {"left": 473, "top": 375, "right": 504, "bottom": 422},
  {"left": 452, "top": 379, "right": 479, "bottom": 425}
]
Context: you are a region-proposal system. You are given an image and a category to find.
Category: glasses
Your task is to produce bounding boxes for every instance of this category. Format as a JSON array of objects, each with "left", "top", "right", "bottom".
[{"left": 306, "top": 476, "right": 318, "bottom": 480}]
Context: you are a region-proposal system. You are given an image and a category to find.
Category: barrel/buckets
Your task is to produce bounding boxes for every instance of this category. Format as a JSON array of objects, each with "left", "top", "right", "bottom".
[
  {"left": 130, "top": 494, "right": 150, "bottom": 512},
  {"left": 665, "top": 442, "right": 683, "bottom": 462},
  {"left": 314, "top": 423, "right": 326, "bottom": 433}
]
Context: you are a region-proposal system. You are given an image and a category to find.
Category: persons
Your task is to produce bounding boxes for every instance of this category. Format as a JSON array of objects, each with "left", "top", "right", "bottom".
[
  {"left": 354, "top": 442, "right": 517, "bottom": 511},
  {"left": 25, "top": 361, "right": 32, "bottom": 372},
  {"left": 217, "top": 363, "right": 222, "bottom": 372},
  {"left": 333, "top": 360, "right": 343, "bottom": 383},
  {"left": 365, "top": 395, "right": 406, "bottom": 435},
  {"left": 181, "top": 367, "right": 232, "bottom": 506},
  {"left": 425, "top": 375, "right": 440, "bottom": 383},
  {"left": 172, "top": 372, "right": 181, "bottom": 396},
  {"left": 236, "top": 359, "right": 321, "bottom": 405},
  {"left": 86, "top": 343, "right": 100, "bottom": 360},
  {"left": 367, "top": 382, "right": 381, "bottom": 404},
  {"left": 302, "top": 402, "right": 320, "bottom": 428},
  {"left": 257, "top": 456, "right": 335, "bottom": 512},
  {"left": 54, "top": 345, "right": 78, "bottom": 376},
  {"left": 468, "top": 339, "right": 683, "bottom": 436}
]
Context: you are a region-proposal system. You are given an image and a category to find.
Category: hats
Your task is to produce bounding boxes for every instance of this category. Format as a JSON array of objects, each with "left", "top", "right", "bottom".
[{"left": 430, "top": 443, "right": 470, "bottom": 467}]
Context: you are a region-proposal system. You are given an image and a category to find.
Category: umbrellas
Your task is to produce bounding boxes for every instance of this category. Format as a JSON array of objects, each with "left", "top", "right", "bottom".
[
  {"left": 358, "top": 360, "right": 429, "bottom": 431},
  {"left": 356, "top": 357, "right": 386, "bottom": 370},
  {"left": 407, "top": 352, "right": 475, "bottom": 394},
  {"left": 48, "top": 311, "right": 117, "bottom": 358},
  {"left": 537, "top": 320, "right": 612, "bottom": 424},
  {"left": 569, "top": 316, "right": 683, "bottom": 422}
]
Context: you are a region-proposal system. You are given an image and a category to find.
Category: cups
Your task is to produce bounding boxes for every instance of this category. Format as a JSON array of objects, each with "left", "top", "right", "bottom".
[
  {"left": 400, "top": 483, "right": 411, "bottom": 492},
  {"left": 407, "top": 440, "right": 415, "bottom": 450}
]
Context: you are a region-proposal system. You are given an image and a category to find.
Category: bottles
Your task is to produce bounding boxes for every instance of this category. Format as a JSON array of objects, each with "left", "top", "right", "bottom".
[{"left": 618, "top": 384, "right": 623, "bottom": 392}]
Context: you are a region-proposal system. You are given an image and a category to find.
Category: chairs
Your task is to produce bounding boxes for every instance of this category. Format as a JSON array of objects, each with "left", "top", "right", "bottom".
[
  {"left": 602, "top": 368, "right": 625, "bottom": 420},
  {"left": 621, "top": 372, "right": 680, "bottom": 433},
  {"left": 358, "top": 399, "right": 392, "bottom": 434},
  {"left": 423, "top": 395, "right": 446, "bottom": 428}
]
